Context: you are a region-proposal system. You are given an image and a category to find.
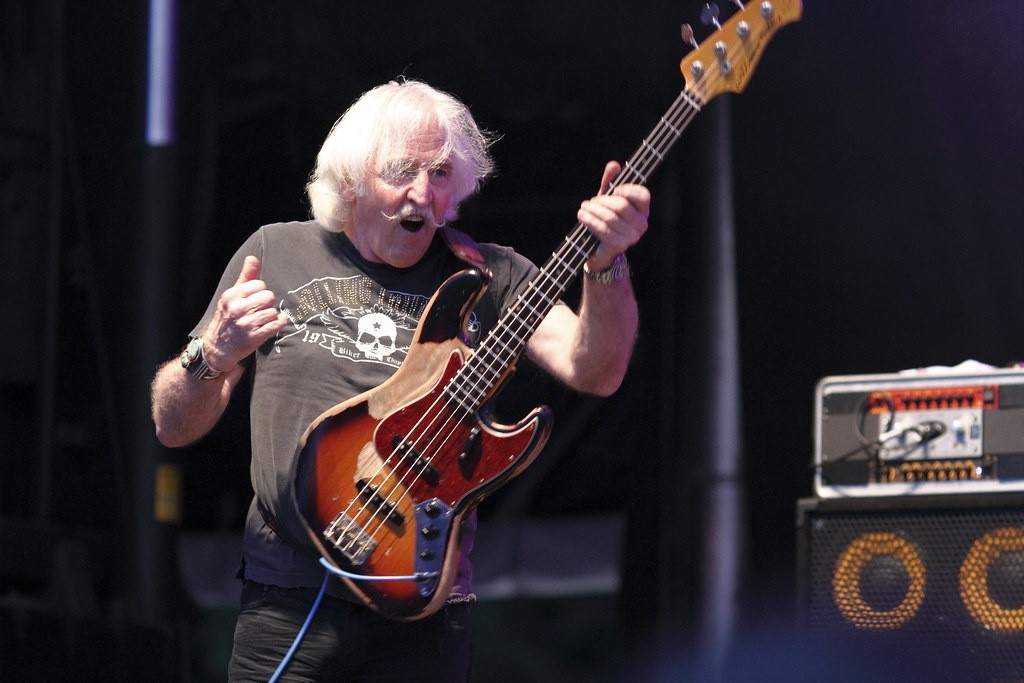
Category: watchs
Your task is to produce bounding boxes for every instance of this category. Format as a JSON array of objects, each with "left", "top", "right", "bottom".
[{"left": 584, "top": 256, "right": 630, "bottom": 286}]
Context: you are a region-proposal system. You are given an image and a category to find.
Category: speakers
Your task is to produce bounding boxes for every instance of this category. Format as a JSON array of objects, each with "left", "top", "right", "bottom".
[{"left": 795, "top": 490, "right": 1024, "bottom": 683}]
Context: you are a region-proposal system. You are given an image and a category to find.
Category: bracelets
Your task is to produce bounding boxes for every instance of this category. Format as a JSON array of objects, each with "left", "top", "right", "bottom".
[{"left": 180, "top": 336, "right": 222, "bottom": 380}]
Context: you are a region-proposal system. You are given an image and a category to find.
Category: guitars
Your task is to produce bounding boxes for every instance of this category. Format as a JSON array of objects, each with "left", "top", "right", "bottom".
[{"left": 288, "top": 0, "right": 802, "bottom": 626}]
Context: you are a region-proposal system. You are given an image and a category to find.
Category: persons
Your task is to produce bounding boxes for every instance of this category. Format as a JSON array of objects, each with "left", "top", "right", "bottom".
[{"left": 151, "top": 81, "right": 651, "bottom": 683}]
